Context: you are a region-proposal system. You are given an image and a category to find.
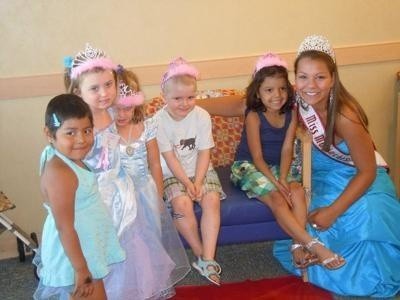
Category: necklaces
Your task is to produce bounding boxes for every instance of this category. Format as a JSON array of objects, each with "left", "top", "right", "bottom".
[{"left": 115, "top": 123, "right": 135, "bottom": 157}]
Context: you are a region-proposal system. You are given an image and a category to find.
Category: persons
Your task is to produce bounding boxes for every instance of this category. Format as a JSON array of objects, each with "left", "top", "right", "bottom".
[
  {"left": 231, "top": 49, "right": 345, "bottom": 271},
  {"left": 271, "top": 34, "right": 400, "bottom": 298},
  {"left": 30, "top": 93, "right": 128, "bottom": 300},
  {"left": 154, "top": 57, "right": 226, "bottom": 289},
  {"left": 111, "top": 62, "right": 165, "bottom": 242},
  {"left": 63, "top": 43, "right": 194, "bottom": 300}
]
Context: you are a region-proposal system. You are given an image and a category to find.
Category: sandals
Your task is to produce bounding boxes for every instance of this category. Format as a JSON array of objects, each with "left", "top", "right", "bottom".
[
  {"left": 190, "top": 254, "right": 222, "bottom": 287},
  {"left": 304, "top": 237, "right": 347, "bottom": 271},
  {"left": 287, "top": 243, "right": 319, "bottom": 269}
]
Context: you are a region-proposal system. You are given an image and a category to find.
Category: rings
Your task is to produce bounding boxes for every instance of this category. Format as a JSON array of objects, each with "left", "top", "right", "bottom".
[{"left": 313, "top": 223, "right": 317, "bottom": 229}]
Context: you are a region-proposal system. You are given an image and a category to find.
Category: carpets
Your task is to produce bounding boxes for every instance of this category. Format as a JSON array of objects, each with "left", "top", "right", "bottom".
[{"left": 164, "top": 275, "right": 333, "bottom": 299}]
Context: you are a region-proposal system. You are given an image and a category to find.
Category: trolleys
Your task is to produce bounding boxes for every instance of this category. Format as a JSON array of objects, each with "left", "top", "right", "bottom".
[{"left": 0, "top": 190, "right": 43, "bottom": 280}]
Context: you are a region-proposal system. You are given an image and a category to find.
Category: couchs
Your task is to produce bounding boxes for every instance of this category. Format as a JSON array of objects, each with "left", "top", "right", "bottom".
[{"left": 133, "top": 87, "right": 305, "bottom": 250}]
